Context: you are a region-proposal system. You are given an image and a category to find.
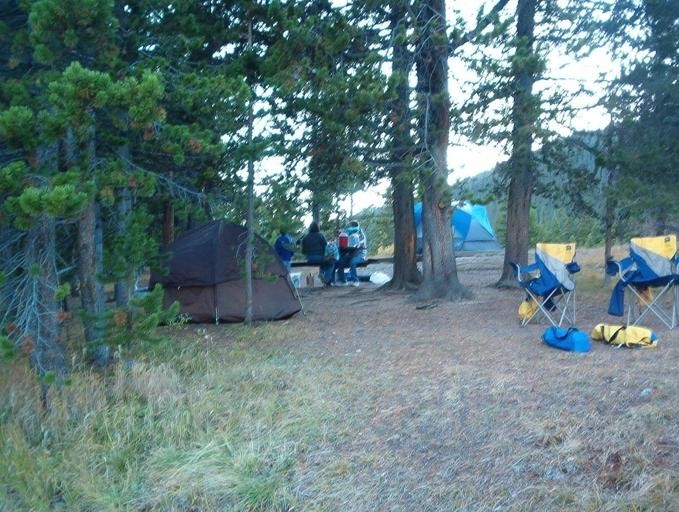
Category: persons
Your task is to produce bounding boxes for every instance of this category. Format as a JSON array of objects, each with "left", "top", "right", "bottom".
[
  {"left": 301, "top": 221, "right": 336, "bottom": 288},
  {"left": 274, "top": 224, "right": 294, "bottom": 272},
  {"left": 335, "top": 220, "right": 367, "bottom": 287}
]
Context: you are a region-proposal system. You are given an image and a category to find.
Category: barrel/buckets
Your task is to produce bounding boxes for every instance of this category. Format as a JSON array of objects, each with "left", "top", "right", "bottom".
[{"left": 339, "top": 233, "right": 348, "bottom": 248}]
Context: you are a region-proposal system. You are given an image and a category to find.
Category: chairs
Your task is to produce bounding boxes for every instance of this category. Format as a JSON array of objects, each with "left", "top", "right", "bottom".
[
  {"left": 512, "top": 243, "right": 576, "bottom": 329},
  {"left": 614, "top": 234, "right": 679, "bottom": 329}
]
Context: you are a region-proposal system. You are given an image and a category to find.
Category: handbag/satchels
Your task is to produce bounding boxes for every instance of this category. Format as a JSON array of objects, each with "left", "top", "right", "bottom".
[
  {"left": 591, "top": 323, "right": 659, "bottom": 349},
  {"left": 542, "top": 325, "right": 591, "bottom": 353}
]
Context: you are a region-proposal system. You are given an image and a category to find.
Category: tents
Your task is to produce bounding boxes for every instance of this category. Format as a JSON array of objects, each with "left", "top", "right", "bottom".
[
  {"left": 413, "top": 198, "right": 501, "bottom": 257},
  {"left": 146, "top": 219, "right": 303, "bottom": 324}
]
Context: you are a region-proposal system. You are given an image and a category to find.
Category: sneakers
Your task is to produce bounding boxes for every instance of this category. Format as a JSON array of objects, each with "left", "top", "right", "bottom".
[
  {"left": 351, "top": 282, "right": 359, "bottom": 287},
  {"left": 335, "top": 281, "right": 349, "bottom": 287}
]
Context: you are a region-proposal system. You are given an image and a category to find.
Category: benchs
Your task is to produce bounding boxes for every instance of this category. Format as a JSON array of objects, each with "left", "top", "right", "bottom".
[{"left": 290, "top": 253, "right": 425, "bottom": 267}]
